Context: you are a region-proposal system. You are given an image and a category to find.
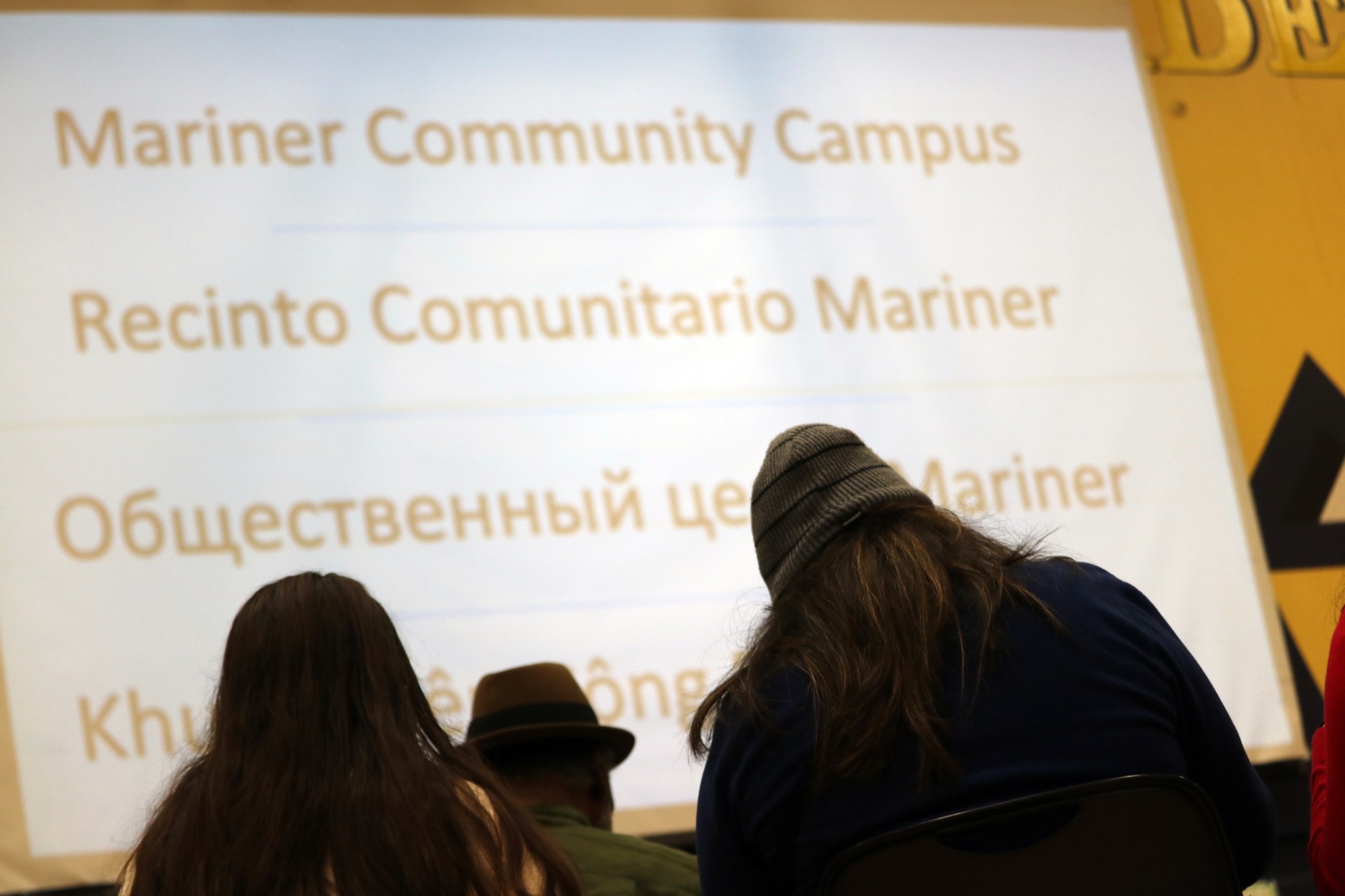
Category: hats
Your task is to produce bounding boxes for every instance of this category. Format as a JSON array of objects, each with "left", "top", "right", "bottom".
[
  {"left": 451, "top": 663, "right": 636, "bottom": 774},
  {"left": 752, "top": 423, "right": 938, "bottom": 604}
]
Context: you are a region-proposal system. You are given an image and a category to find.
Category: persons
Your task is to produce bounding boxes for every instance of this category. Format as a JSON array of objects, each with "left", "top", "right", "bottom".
[
  {"left": 119, "top": 574, "right": 596, "bottom": 896},
  {"left": 1307, "top": 601, "right": 1345, "bottom": 896},
  {"left": 456, "top": 663, "right": 702, "bottom": 896},
  {"left": 691, "top": 425, "right": 1278, "bottom": 896}
]
